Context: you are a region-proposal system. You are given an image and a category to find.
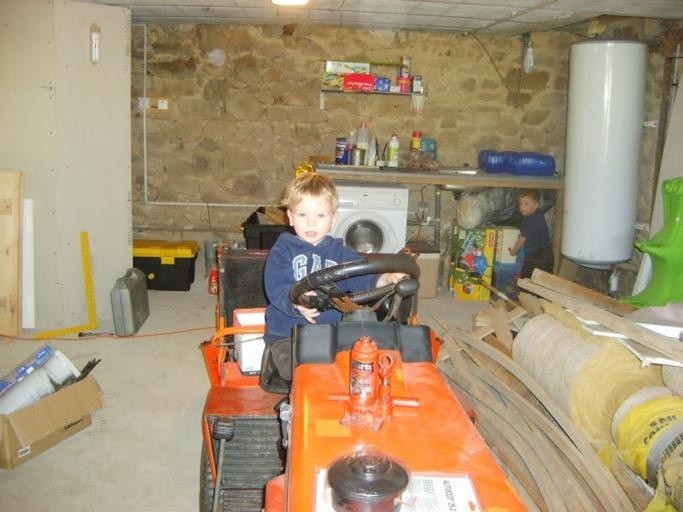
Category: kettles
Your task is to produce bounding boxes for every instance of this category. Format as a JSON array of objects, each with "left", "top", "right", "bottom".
[{"left": 346, "top": 131, "right": 356, "bottom": 165}]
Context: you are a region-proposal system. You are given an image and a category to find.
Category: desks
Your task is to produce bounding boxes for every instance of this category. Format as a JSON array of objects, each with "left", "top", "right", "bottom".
[
  {"left": 281, "top": 359, "right": 529, "bottom": 512},
  {"left": 319, "top": 163, "right": 565, "bottom": 275}
]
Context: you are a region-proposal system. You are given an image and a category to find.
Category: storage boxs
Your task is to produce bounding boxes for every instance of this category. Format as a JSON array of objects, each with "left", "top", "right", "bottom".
[
  {"left": 108, "top": 268, "right": 148, "bottom": 340},
  {"left": 0, "top": 375, "right": 103, "bottom": 471},
  {"left": 131, "top": 237, "right": 200, "bottom": 293}
]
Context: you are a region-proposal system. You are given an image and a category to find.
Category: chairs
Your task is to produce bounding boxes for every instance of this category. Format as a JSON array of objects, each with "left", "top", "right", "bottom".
[{"left": 217, "top": 246, "right": 420, "bottom": 374}]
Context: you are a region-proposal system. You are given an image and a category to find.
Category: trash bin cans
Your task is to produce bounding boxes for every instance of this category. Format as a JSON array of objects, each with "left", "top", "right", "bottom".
[{"left": 414, "top": 247, "right": 440, "bottom": 298}]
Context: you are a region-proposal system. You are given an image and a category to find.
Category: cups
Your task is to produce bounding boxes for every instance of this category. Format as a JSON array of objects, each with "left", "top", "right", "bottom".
[
  {"left": 349, "top": 148, "right": 365, "bottom": 166},
  {"left": 205, "top": 259, "right": 217, "bottom": 278},
  {"left": 204, "top": 237, "right": 218, "bottom": 258}
]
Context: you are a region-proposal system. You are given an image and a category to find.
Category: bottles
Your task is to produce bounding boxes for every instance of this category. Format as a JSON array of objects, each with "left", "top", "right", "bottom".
[
  {"left": 445, "top": 218, "right": 486, "bottom": 297},
  {"left": 216, "top": 240, "right": 239, "bottom": 264},
  {"left": 386, "top": 134, "right": 399, "bottom": 168},
  {"left": 348, "top": 334, "right": 379, "bottom": 408},
  {"left": 355, "top": 120, "right": 370, "bottom": 166},
  {"left": 410, "top": 130, "right": 421, "bottom": 150},
  {"left": 207, "top": 265, "right": 218, "bottom": 295}
]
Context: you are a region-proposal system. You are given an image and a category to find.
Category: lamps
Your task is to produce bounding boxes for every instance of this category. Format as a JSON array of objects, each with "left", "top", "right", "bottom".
[{"left": 522, "top": 38, "right": 535, "bottom": 74}]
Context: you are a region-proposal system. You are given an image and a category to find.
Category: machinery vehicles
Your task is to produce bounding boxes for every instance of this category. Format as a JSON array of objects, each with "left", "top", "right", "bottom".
[{"left": 199, "top": 223, "right": 532, "bottom": 512}]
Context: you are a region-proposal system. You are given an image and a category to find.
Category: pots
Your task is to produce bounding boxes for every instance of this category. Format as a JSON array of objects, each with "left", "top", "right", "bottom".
[{"left": 326, "top": 454, "right": 408, "bottom": 511}]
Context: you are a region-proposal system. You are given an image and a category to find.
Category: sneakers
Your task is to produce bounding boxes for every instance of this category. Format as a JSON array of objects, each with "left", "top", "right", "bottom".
[{"left": 259, "top": 342, "right": 289, "bottom": 394}]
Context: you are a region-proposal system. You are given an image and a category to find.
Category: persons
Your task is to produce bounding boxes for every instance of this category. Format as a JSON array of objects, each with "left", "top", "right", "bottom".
[
  {"left": 261, "top": 174, "right": 411, "bottom": 383},
  {"left": 511, "top": 188, "right": 555, "bottom": 301}
]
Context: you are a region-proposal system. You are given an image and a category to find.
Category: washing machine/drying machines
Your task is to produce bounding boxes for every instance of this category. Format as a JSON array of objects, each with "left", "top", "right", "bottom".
[{"left": 325, "top": 180, "right": 410, "bottom": 255}]
